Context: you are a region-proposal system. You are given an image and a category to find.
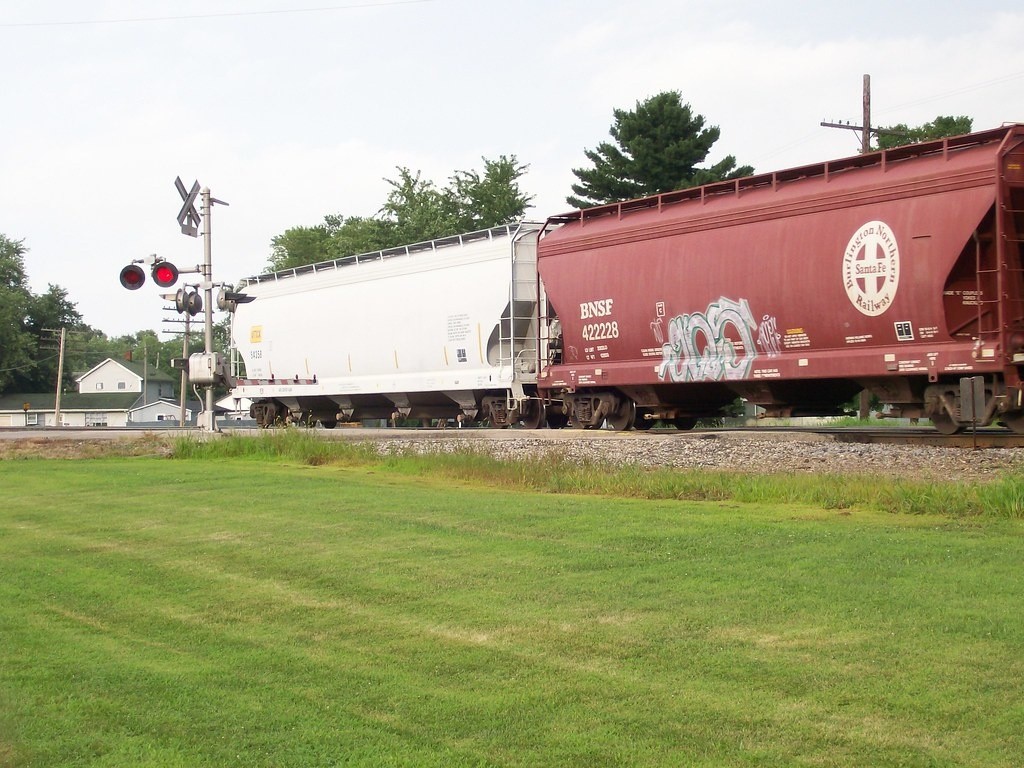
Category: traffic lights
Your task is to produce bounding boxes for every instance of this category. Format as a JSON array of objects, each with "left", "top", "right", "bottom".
[
  {"left": 217, "top": 291, "right": 257, "bottom": 313},
  {"left": 188, "top": 292, "right": 203, "bottom": 318},
  {"left": 160, "top": 288, "right": 189, "bottom": 313},
  {"left": 119, "top": 266, "right": 145, "bottom": 289},
  {"left": 152, "top": 262, "right": 179, "bottom": 287}
]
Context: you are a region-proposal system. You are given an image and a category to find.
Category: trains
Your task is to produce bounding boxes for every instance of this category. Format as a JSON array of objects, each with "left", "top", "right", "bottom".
[{"left": 230, "top": 124, "right": 1024, "bottom": 438}]
